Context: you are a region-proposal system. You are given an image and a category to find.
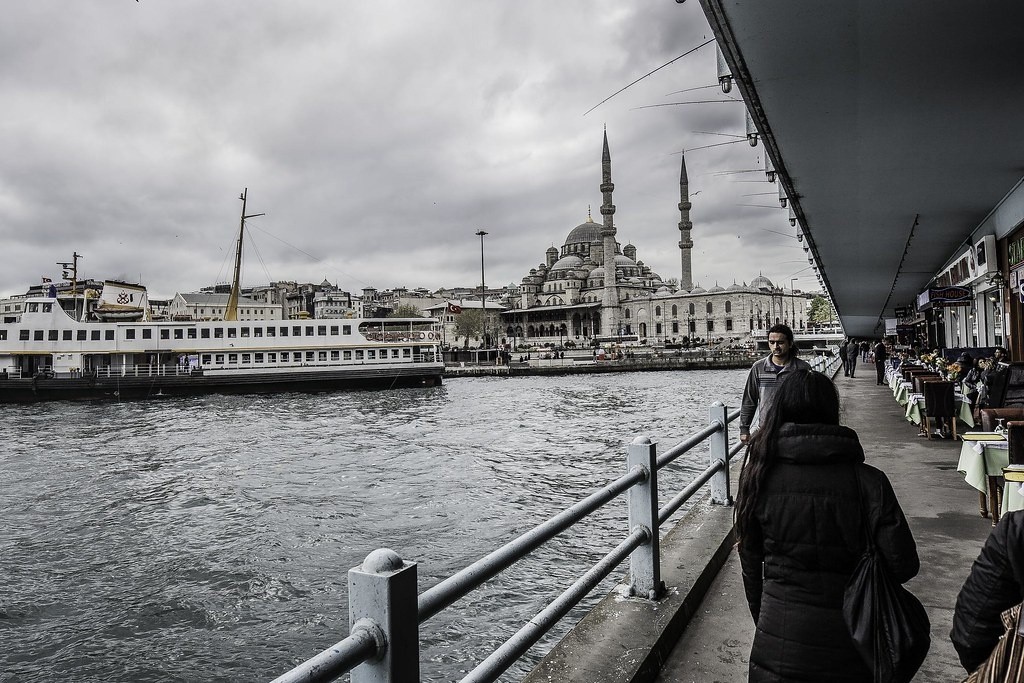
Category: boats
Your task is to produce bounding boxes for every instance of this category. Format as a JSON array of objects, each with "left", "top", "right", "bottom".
[
  {"left": 93, "top": 308, "right": 145, "bottom": 323},
  {"left": 0, "top": 186, "right": 447, "bottom": 397}
]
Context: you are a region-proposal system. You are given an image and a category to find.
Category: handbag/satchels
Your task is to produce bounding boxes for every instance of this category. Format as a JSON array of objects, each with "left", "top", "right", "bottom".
[
  {"left": 960, "top": 599, "right": 1024, "bottom": 683},
  {"left": 840, "top": 547, "right": 931, "bottom": 683}
]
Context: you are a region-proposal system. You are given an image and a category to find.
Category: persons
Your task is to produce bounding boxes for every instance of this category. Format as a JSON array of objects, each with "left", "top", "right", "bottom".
[
  {"left": 593, "top": 351, "right": 596, "bottom": 356},
  {"left": 739, "top": 324, "right": 811, "bottom": 445},
  {"left": 858, "top": 340, "right": 870, "bottom": 363},
  {"left": 554, "top": 350, "right": 564, "bottom": 359},
  {"left": 733, "top": 369, "right": 919, "bottom": 683},
  {"left": 932, "top": 345, "right": 947, "bottom": 357},
  {"left": 520, "top": 353, "right": 530, "bottom": 363},
  {"left": 896, "top": 353, "right": 912, "bottom": 372},
  {"left": 956, "top": 348, "right": 1011, "bottom": 388},
  {"left": 838, "top": 342, "right": 850, "bottom": 377},
  {"left": 950, "top": 510, "right": 1024, "bottom": 676},
  {"left": 627, "top": 351, "right": 634, "bottom": 358},
  {"left": 875, "top": 337, "right": 888, "bottom": 385},
  {"left": 846, "top": 338, "right": 858, "bottom": 378},
  {"left": 685, "top": 341, "right": 689, "bottom": 351}
]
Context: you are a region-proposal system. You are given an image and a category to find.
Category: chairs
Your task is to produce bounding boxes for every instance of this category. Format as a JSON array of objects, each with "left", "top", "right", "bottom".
[
  {"left": 915, "top": 376, "right": 942, "bottom": 393},
  {"left": 1006, "top": 420, "right": 1024, "bottom": 465},
  {"left": 920, "top": 380, "right": 957, "bottom": 440},
  {"left": 979, "top": 408, "right": 1024, "bottom": 432},
  {"left": 901, "top": 367, "right": 923, "bottom": 379},
  {"left": 905, "top": 371, "right": 911, "bottom": 382},
  {"left": 911, "top": 371, "right": 941, "bottom": 392}
]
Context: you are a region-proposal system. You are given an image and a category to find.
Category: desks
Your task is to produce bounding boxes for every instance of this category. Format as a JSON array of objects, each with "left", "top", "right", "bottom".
[{"left": 883, "top": 359, "right": 1024, "bottom": 529}]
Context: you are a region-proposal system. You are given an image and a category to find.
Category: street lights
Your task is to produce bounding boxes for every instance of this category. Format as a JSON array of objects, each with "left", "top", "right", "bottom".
[
  {"left": 790, "top": 278, "right": 799, "bottom": 334},
  {"left": 475, "top": 228, "right": 489, "bottom": 350}
]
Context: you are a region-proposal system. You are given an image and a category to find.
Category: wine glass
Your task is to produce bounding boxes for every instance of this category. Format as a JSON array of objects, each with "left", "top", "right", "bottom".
[{"left": 994, "top": 418, "right": 1005, "bottom": 433}]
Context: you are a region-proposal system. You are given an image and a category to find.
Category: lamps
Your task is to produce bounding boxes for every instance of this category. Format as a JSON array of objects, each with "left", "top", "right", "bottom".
[
  {"left": 720, "top": 75, "right": 840, "bottom": 323},
  {"left": 874, "top": 214, "right": 920, "bottom": 334},
  {"left": 989, "top": 271, "right": 1008, "bottom": 290}
]
